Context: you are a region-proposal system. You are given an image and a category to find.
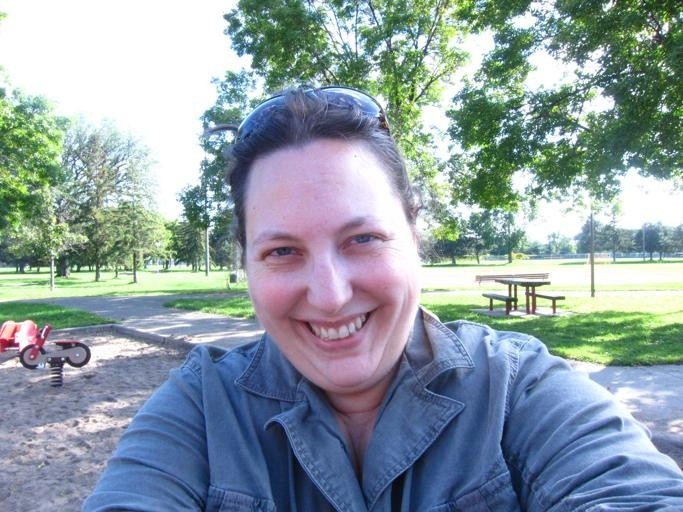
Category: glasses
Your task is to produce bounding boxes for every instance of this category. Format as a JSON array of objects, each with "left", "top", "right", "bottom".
[{"left": 234, "top": 85, "right": 390, "bottom": 145}]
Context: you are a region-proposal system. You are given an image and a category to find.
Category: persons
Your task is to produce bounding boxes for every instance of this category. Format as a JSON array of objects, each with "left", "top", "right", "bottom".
[{"left": 81, "top": 84, "right": 681, "bottom": 511}]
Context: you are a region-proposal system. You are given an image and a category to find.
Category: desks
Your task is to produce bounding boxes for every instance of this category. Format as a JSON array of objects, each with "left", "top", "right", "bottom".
[{"left": 495, "top": 278, "right": 550, "bottom": 314}]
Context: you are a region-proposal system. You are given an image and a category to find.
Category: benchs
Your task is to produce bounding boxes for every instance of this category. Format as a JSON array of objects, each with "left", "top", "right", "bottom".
[
  {"left": 525, "top": 292, "right": 565, "bottom": 313},
  {"left": 484, "top": 292, "right": 518, "bottom": 314},
  {"left": 475, "top": 274, "right": 550, "bottom": 284}
]
